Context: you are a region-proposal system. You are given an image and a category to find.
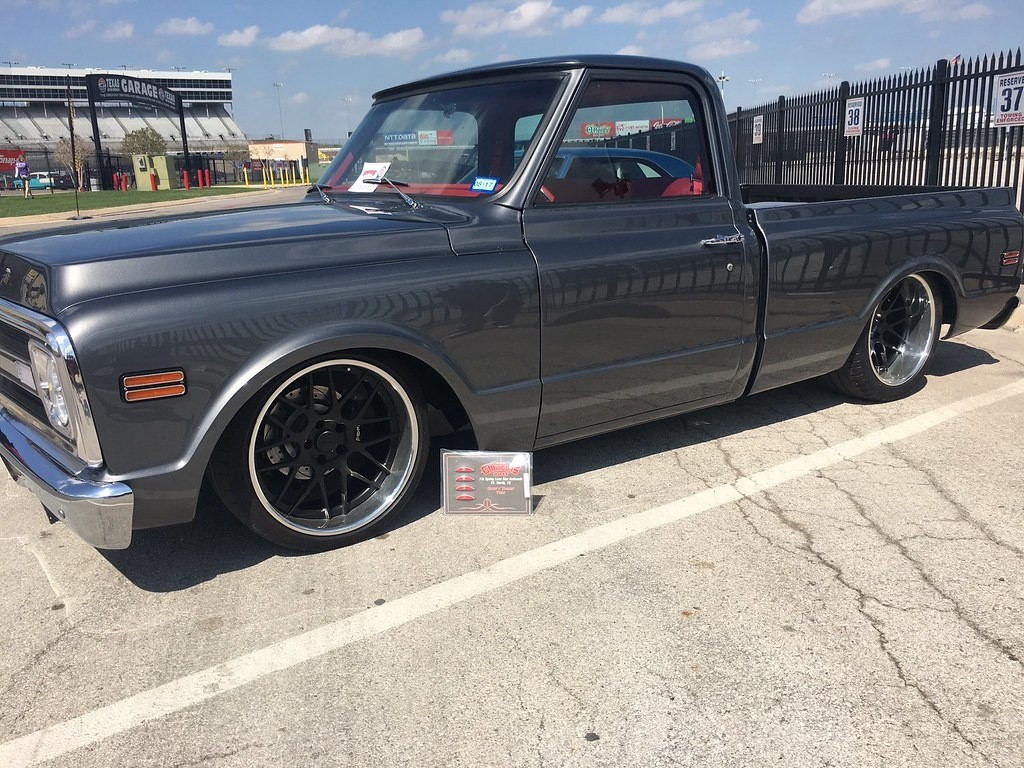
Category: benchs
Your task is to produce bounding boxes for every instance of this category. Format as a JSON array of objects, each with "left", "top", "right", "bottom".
[{"left": 541, "top": 177, "right": 703, "bottom": 203}]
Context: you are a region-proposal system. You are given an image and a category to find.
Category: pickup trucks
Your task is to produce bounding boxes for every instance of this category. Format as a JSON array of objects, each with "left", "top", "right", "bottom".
[{"left": 0, "top": 53, "right": 1024, "bottom": 554}]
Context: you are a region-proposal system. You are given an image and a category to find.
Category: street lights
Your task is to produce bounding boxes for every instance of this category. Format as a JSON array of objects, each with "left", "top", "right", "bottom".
[
  {"left": 717, "top": 69, "right": 730, "bottom": 106},
  {"left": 272, "top": 82, "right": 285, "bottom": 140}
]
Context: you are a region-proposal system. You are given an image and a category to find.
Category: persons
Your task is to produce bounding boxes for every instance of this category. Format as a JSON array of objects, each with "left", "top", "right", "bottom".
[
  {"left": 117, "top": 168, "right": 123, "bottom": 189},
  {"left": 15, "top": 154, "right": 34, "bottom": 199},
  {"left": 129, "top": 168, "right": 135, "bottom": 187}
]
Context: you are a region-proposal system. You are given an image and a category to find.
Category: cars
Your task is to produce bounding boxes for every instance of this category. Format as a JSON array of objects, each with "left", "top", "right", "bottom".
[
  {"left": 0, "top": 171, "right": 74, "bottom": 191},
  {"left": 453, "top": 147, "right": 708, "bottom": 208}
]
